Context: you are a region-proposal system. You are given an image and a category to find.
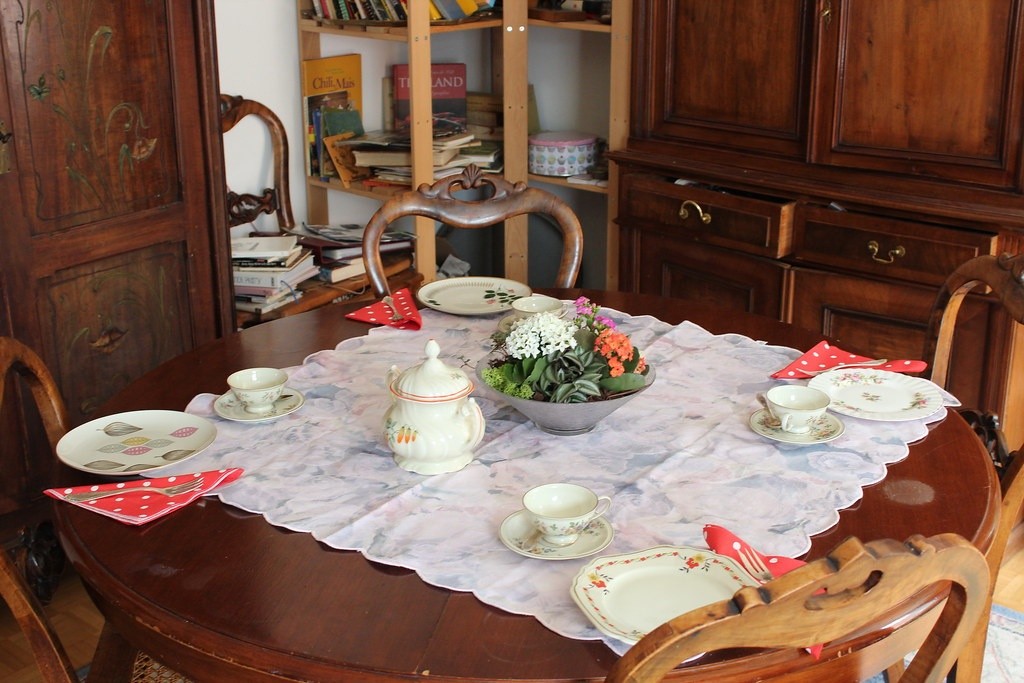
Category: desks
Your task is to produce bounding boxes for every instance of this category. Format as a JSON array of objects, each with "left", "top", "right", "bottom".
[{"left": 55, "top": 290, "right": 999, "bottom": 683}]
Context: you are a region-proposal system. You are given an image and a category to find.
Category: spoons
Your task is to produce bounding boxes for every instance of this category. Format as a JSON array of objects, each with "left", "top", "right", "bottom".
[
  {"left": 382, "top": 295, "right": 403, "bottom": 321},
  {"left": 755, "top": 393, "right": 781, "bottom": 429}
]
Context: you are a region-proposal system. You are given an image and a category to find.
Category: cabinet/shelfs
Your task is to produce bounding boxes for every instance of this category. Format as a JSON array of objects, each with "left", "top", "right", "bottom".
[
  {"left": 0, "top": 0, "right": 236, "bottom": 604},
  {"left": 296, "top": 0, "right": 1023, "bottom": 443}
]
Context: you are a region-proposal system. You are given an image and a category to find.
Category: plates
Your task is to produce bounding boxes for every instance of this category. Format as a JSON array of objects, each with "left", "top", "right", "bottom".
[
  {"left": 497, "top": 508, "right": 614, "bottom": 560},
  {"left": 56, "top": 409, "right": 217, "bottom": 475},
  {"left": 214, "top": 387, "right": 304, "bottom": 422},
  {"left": 570, "top": 545, "right": 763, "bottom": 651},
  {"left": 749, "top": 407, "right": 845, "bottom": 446},
  {"left": 417, "top": 277, "right": 533, "bottom": 317},
  {"left": 805, "top": 367, "right": 943, "bottom": 421}
]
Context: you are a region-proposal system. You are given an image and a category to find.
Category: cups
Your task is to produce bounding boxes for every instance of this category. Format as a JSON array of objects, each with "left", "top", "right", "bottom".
[
  {"left": 511, "top": 295, "right": 569, "bottom": 320},
  {"left": 384, "top": 339, "right": 486, "bottom": 474},
  {"left": 227, "top": 367, "right": 290, "bottom": 412},
  {"left": 522, "top": 482, "right": 612, "bottom": 544},
  {"left": 766, "top": 384, "right": 830, "bottom": 434}
]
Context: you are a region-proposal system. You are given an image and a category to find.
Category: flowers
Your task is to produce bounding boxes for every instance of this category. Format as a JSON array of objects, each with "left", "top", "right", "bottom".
[{"left": 483, "top": 295, "right": 648, "bottom": 405}]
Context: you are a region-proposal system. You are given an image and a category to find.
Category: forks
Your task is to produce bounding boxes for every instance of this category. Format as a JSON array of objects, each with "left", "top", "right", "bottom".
[{"left": 796, "top": 358, "right": 887, "bottom": 377}]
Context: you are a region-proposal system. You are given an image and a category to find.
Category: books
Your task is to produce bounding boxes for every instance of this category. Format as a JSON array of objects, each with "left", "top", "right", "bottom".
[
  {"left": 302, "top": 53, "right": 608, "bottom": 189},
  {"left": 249, "top": 223, "right": 416, "bottom": 284},
  {"left": 231, "top": 236, "right": 321, "bottom": 315},
  {"left": 313, "top": 0, "right": 602, "bottom": 22}
]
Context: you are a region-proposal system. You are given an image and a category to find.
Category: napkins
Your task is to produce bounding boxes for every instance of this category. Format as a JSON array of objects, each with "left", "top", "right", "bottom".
[
  {"left": 344, "top": 288, "right": 422, "bottom": 330},
  {"left": 772, "top": 340, "right": 926, "bottom": 380},
  {"left": 703, "top": 524, "right": 828, "bottom": 660},
  {"left": 42, "top": 467, "right": 245, "bottom": 526}
]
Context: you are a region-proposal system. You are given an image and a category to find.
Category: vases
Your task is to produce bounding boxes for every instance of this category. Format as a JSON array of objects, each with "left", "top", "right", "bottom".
[{"left": 475, "top": 352, "right": 655, "bottom": 435}]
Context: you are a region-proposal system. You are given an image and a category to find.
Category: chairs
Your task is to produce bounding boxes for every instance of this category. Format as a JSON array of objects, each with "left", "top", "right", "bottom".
[
  {"left": 220, "top": 93, "right": 415, "bottom": 333},
  {"left": 363, "top": 162, "right": 586, "bottom": 297},
  {"left": 882, "top": 253, "right": 1024, "bottom": 683},
  {"left": 0, "top": 335, "right": 191, "bottom": 683},
  {"left": 604, "top": 533, "right": 989, "bottom": 683}
]
